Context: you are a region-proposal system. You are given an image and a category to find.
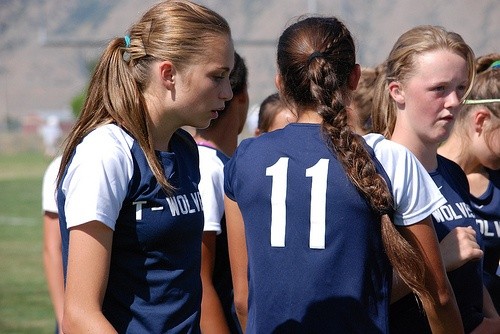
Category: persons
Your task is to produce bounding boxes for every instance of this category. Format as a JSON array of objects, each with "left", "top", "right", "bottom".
[
  {"left": 191, "top": 51, "right": 249, "bottom": 332},
  {"left": 42, "top": 155, "right": 64, "bottom": 334},
  {"left": 224, "top": 16, "right": 465, "bottom": 334},
  {"left": 55, "top": 0, "right": 236, "bottom": 334},
  {"left": 435, "top": 53, "right": 500, "bottom": 334},
  {"left": 372, "top": 24, "right": 500, "bottom": 334}
]
